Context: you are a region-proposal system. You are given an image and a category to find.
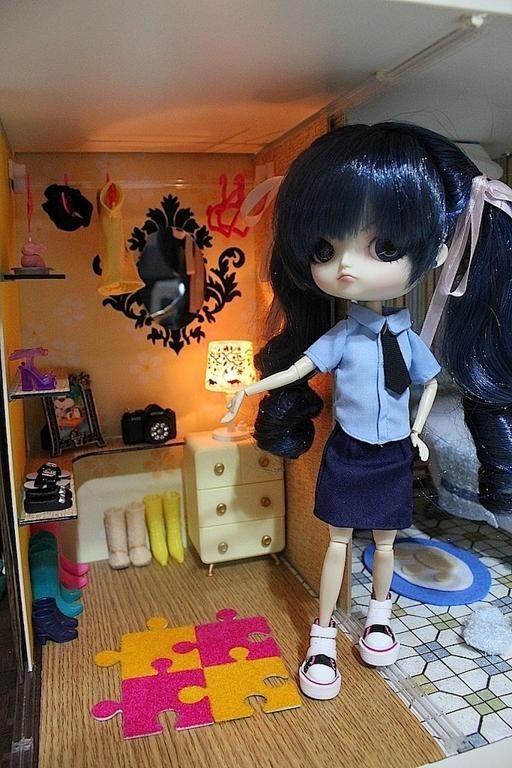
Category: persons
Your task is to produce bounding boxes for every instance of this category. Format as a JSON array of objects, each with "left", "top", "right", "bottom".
[{"left": 219, "top": 121, "right": 512, "bottom": 767}]
